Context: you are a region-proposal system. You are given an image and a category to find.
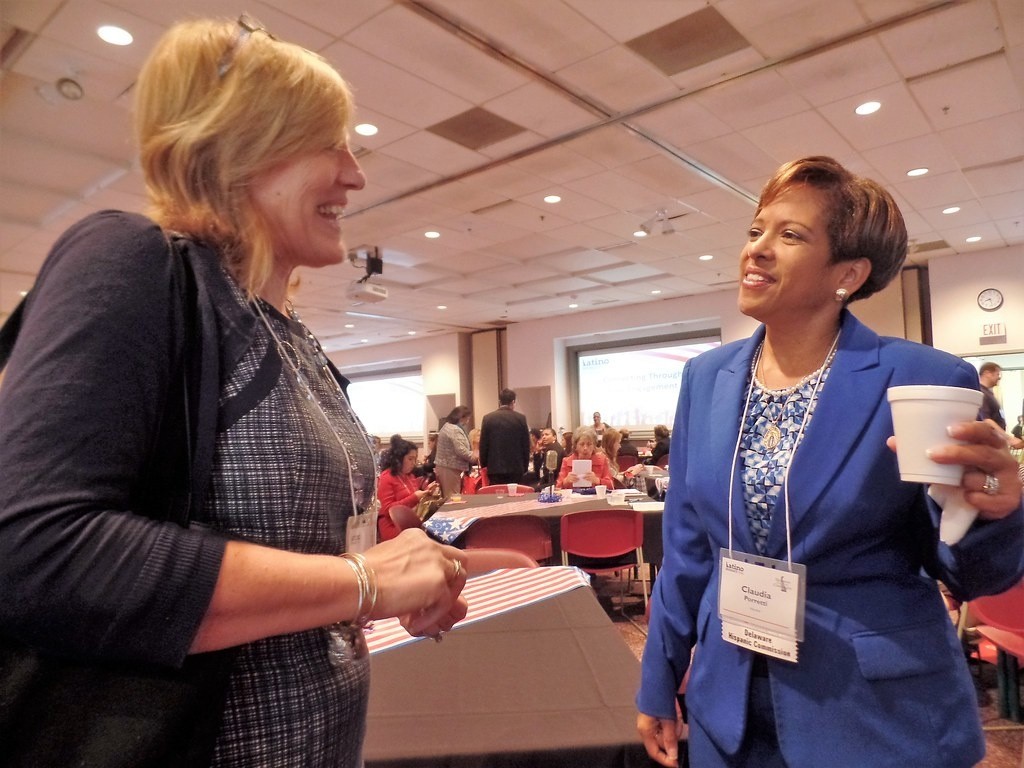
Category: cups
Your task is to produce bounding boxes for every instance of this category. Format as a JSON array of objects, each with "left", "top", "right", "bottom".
[
  {"left": 452, "top": 493, "right": 462, "bottom": 502},
  {"left": 645, "top": 451, "right": 650, "bottom": 455},
  {"left": 887, "top": 385, "right": 984, "bottom": 486},
  {"left": 496, "top": 489, "right": 505, "bottom": 498},
  {"left": 595, "top": 486, "right": 606, "bottom": 496},
  {"left": 611, "top": 490, "right": 624, "bottom": 503},
  {"left": 507, "top": 483, "right": 517, "bottom": 496}
]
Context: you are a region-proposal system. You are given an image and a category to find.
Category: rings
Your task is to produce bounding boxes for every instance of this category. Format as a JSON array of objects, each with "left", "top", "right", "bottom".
[
  {"left": 984, "top": 475, "right": 1000, "bottom": 496},
  {"left": 448, "top": 558, "right": 462, "bottom": 587}
]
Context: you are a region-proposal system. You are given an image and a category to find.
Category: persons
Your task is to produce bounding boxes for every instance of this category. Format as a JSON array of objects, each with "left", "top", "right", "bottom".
[
  {"left": 635, "top": 157, "right": 1024, "bottom": 768},
  {"left": 376, "top": 439, "right": 440, "bottom": 542},
  {"left": 479, "top": 388, "right": 531, "bottom": 485},
  {"left": 0, "top": 18, "right": 467, "bottom": 767},
  {"left": 556, "top": 425, "right": 614, "bottom": 490},
  {"left": 433, "top": 405, "right": 480, "bottom": 498},
  {"left": 643, "top": 424, "right": 671, "bottom": 465},
  {"left": 531, "top": 412, "right": 638, "bottom": 492},
  {"left": 372, "top": 429, "right": 480, "bottom": 471},
  {"left": 977, "top": 362, "right": 1024, "bottom": 441}
]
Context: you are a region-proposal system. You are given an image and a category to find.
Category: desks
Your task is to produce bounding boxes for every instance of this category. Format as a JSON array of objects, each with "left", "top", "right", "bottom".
[{"left": 424, "top": 487, "right": 664, "bottom": 591}]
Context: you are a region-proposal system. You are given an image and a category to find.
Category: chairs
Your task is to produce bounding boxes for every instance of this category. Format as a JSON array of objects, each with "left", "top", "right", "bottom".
[{"left": 361, "top": 449, "right": 1024, "bottom": 726}]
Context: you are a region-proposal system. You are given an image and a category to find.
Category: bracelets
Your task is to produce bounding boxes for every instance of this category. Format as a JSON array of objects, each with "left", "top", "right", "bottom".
[{"left": 322, "top": 551, "right": 378, "bottom": 666}]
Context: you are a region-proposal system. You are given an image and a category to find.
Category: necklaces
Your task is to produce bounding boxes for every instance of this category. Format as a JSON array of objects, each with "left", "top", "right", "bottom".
[
  {"left": 751, "top": 341, "right": 837, "bottom": 396},
  {"left": 760, "top": 354, "right": 827, "bottom": 449}
]
